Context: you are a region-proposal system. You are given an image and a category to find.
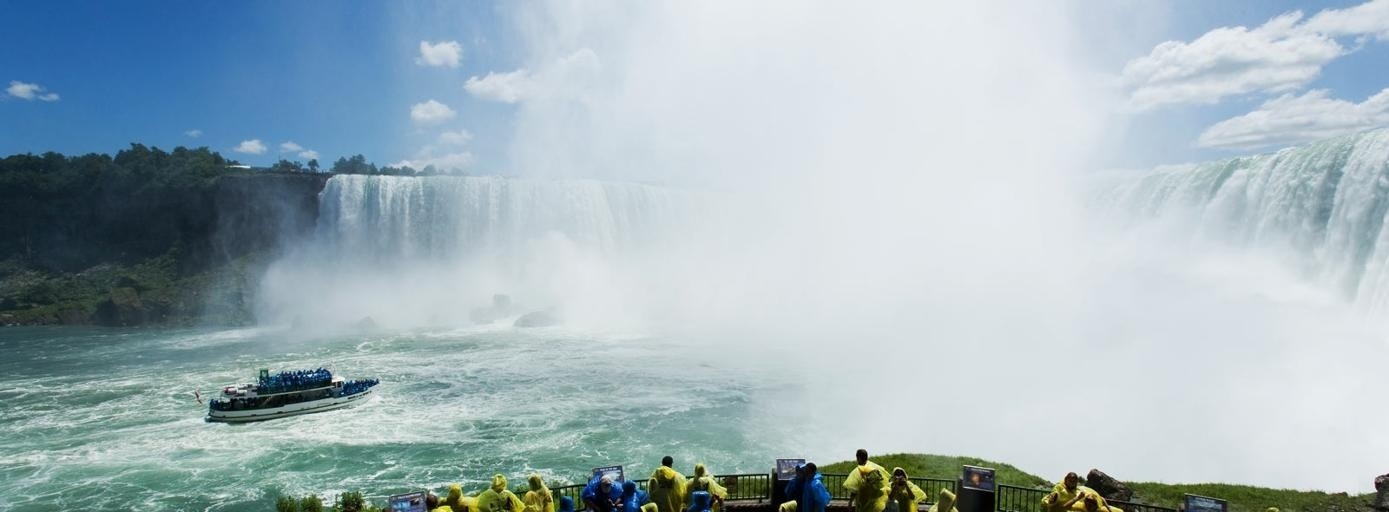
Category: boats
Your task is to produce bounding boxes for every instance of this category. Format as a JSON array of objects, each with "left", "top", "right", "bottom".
[{"left": 203, "top": 368, "right": 383, "bottom": 426}]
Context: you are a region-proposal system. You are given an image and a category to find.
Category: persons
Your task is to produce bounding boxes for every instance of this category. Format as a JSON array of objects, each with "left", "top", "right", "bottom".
[
  {"left": 1040, "top": 472, "right": 1123, "bottom": 512},
  {"left": 424, "top": 472, "right": 555, "bottom": 511},
  {"left": 784, "top": 462, "right": 830, "bottom": 511},
  {"left": 559, "top": 496, "right": 575, "bottom": 511},
  {"left": 929, "top": 488, "right": 959, "bottom": 512},
  {"left": 843, "top": 449, "right": 927, "bottom": 512},
  {"left": 582, "top": 456, "right": 727, "bottom": 512},
  {"left": 210, "top": 367, "right": 380, "bottom": 411}
]
